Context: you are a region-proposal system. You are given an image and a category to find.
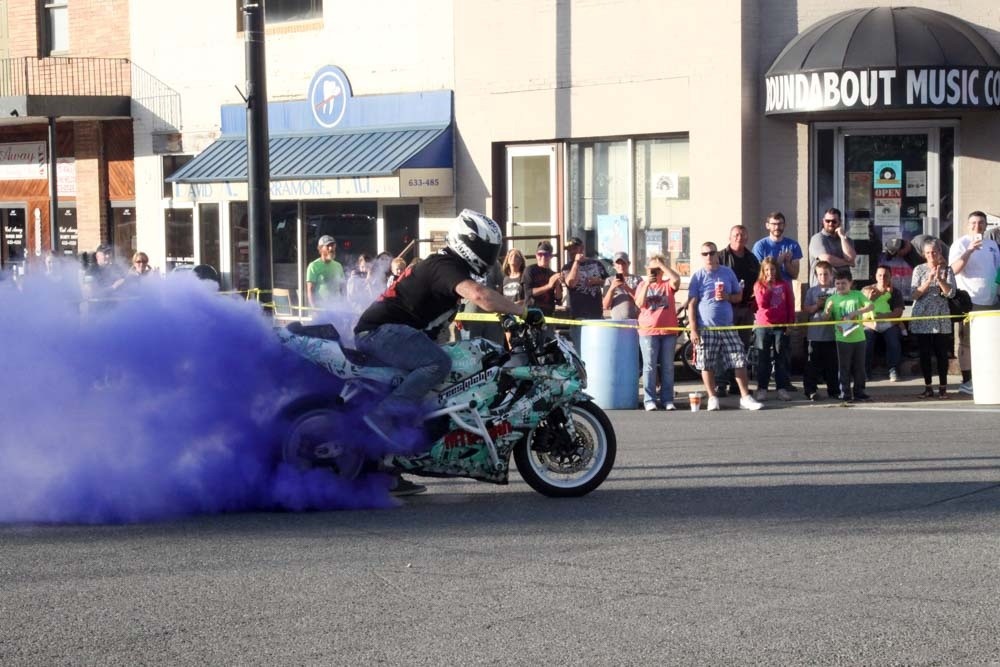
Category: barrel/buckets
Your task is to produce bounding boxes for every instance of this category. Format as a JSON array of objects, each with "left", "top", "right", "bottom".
[{"left": 579, "top": 318, "right": 640, "bottom": 410}]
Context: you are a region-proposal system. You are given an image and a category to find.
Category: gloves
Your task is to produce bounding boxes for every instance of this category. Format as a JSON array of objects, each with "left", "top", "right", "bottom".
[{"left": 522, "top": 306, "right": 545, "bottom": 329}]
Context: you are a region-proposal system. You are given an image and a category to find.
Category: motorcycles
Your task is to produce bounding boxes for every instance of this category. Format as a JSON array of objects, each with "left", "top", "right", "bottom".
[{"left": 280, "top": 270, "right": 618, "bottom": 507}]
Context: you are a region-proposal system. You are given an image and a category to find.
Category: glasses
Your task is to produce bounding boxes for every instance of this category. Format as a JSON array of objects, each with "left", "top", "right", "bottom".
[
  {"left": 135, "top": 260, "right": 146, "bottom": 264},
  {"left": 536, "top": 251, "right": 551, "bottom": 257},
  {"left": 702, "top": 250, "right": 717, "bottom": 257},
  {"left": 825, "top": 219, "right": 837, "bottom": 224}
]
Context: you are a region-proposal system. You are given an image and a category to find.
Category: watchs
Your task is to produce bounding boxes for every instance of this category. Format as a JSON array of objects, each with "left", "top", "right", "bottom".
[{"left": 766, "top": 279, "right": 775, "bottom": 283}]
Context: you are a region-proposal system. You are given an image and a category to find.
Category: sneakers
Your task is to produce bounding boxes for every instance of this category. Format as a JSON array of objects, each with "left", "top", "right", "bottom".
[
  {"left": 708, "top": 396, "right": 719, "bottom": 410},
  {"left": 739, "top": 395, "right": 764, "bottom": 410}
]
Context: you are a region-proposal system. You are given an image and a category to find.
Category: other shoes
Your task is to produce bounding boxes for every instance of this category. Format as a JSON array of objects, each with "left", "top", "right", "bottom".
[
  {"left": 776, "top": 388, "right": 792, "bottom": 401},
  {"left": 387, "top": 476, "right": 427, "bottom": 496},
  {"left": 644, "top": 402, "right": 657, "bottom": 411},
  {"left": 842, "top": 392, "right": 854, "bottom": 404},
  {"left": 852, "top": 391, "right": 870, "bottom": 402},
  {"left": 889, "top": 367, "right": 901, "bottom": 381},
  {"left": 664, "top": 402, "right": 676, "bottom": 411},
  {"left": 807, "top": 391, "right": 820, "bottom": 402},
  {"left": 958, "top": 381, "right": 973, "bottom": 396},
  {"left": 918, "top": 386, "right": 934, "bottom": 398},
  {"left": 938, "top": 386, "right": 948, "bottom": 399},
  {"left": 756, "top": 389, "right": 767, "bottom": 400},
  {"left": 364, "top": 410, "right": 408, "bottom": 449},
  {"left": 827, "top": 390, "right": 842, "bottom": 399}
]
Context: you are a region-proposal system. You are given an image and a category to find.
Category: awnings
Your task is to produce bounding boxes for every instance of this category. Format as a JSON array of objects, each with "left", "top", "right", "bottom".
[
  {"left": 763, "top": 5, "right": 1000, "bottom": 119},
  {"left": 164, "top": 122, "right": 454, "bottom": 203}
]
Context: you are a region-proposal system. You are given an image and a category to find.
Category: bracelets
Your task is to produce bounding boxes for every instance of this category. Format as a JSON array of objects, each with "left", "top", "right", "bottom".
[
  {"left": 854, "top": 310, "right": 858, "bottom": 315},
  {"left": 840, "top": 235, "right": 848, "bottom": 239},
  {"left": 936, "top": 279, "right": 941, "bottom": 282}
]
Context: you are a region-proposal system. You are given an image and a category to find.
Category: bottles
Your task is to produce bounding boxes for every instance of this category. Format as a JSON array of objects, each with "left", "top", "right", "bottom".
[{"left": 739, "top": 279, "right": 745, "bottom": 288}]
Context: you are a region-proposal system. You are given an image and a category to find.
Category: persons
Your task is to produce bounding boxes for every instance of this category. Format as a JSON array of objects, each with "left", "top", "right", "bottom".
[
  {"left": 633, "top": 255, "right": 680, "bottom": 411},
  {"left": 45, "top": 252, "right": 66, "bottom": 283},
  {"left": 82, "top": 241, "right": 127, "bottom": 318},
  {"left": 518, "top": 240, "right": 563, "bottom": 318},
  {"left": 947, "top": 211, "right": 1000, "bottom": 397},
  {"left": 859, "top": 265, "right": 908, "bottom": 383},
  {"left": 750, "top": 210, "right": 803, "bottom": 391},
  {"left": 909, "top": 238, "right": 957, "bottom": 400},
  {"left": 821, "top": 270, "right": 875, "bottom": 402},
  {"left": 501, "top": 248, "right": 526, "bottom": 303},
  {"left": 602, "top": 252, "right": 644, "bottom": 321},
  {"left": 886, "top": 232, "right": 950, "bottom": 267},
  {"left": 687, "top": 242, "right": 765, "bottom": 412},
  {"left": 801, "top": 259, "right": 844, "bottom": 402},
  {"left": 123, "top": 251, "right": 161, "bottom": 298},
  {"left": 563, "top": 237, "right": 610, "bottom": 357},
  {"left": 808, "top": 208, "right": 857, "bottom": 289},
  {"left": 716, "top": 223, "right": 761, "bottom": 396},
  {"left": 306, "top": 236, "right": 346, "bottom": 326},
  {"left": 752, "top": 256, "right": 792, "bottom": 400},
  {"left": 345, "top": 251, "right": 424, "bottom": 313},
  {"left": 354, "top": 207, "right": 546, "bottom": 499}
]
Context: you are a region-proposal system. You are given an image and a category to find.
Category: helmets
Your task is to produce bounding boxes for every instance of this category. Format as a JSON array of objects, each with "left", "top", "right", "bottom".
[{"left": 446, "top": 208, "right": 501, "bottom": 278}]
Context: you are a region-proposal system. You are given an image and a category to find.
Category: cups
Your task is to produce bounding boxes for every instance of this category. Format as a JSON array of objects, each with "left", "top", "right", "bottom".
[
  {"left": 714, "top": 282, "right": 725, "bottom": 301},
  {"left": 689, "top": 393, "right": 701, "bottom": 412}
]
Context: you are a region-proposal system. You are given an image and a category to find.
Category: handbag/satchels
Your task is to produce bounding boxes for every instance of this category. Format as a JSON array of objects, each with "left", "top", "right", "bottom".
[{"left": 944, "top": 267, "right": 973, "bottom": 322}]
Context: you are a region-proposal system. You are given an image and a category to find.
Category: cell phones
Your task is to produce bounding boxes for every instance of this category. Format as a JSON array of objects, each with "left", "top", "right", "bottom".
[
  {"left": 651, "top": 269, "right": 657, "bottom": 278},
  {"left": 615, "top": 274, "right": 624, "bottom": 283},
  {"left": 974, "top": 234, "right": 983, "bottom": 249}
]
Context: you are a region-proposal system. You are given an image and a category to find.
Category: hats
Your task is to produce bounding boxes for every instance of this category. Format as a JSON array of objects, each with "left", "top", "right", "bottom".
[
  {"left": 884, "top": 237, "right": 902, "bottom": 258},
  {"left": 614, "top": 252, "right": 629, "bottom": 262},
  {"left": 96, "top": 245, "right": 112, "bottom": 253},
  {"left": 318, "top": 235, "right": 335, "bottom": 246},
  {"left": 564, "top": 237, "right": 583, "bottom": 250}
]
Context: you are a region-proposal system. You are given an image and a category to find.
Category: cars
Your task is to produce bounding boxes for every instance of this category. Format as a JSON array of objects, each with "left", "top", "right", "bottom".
[{"left": 171, "top": 264, "right": 219, "bottom": 283}]
